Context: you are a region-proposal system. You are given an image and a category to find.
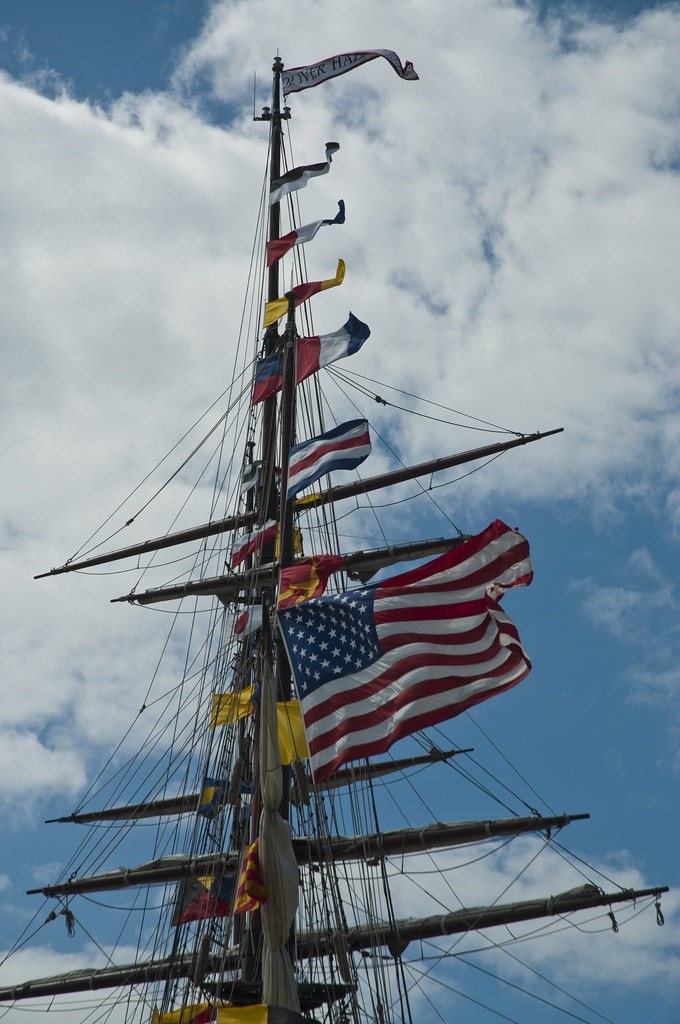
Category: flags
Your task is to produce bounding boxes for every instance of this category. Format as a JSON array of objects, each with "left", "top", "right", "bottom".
[
  {"left": 256, "top": 528, "right": 304, "bottom": 565},
  {"left": 253, "top": 311, "right": 371, "bottom": 406},
  {"left": 277, "top": 702, "right": 311, "bottom": 765},
  {"left": 266, "top": 199, "right": 345, "bottom": 267},
  {"left": 276, "top": 554, "right": 343, "bottom": 611},
  {"left": 268, "top": 141, "right": 340, "bottom": 207},
  {"left": 211, "top": 685, "right": 257, "bottom": 728},
  {"left": 276, "top": 518, "right": 535, "bottom": 789},
  {"left": 217, "top": 1004, "right": 321, "bottom": 1024},
  {"left": 263, "top": 259, "right": 345, "bottom": 329},
  {"left": 240, "top": 418, "right": 372, "bottom": 503},
  {"left": 151, "top": 1000, "right": 232, "bottom": 1024},
  {"left": 282, "top": 50, "right": 420, "bottom": 95},
  {"left": 231, "top": 836, "right": 264, "bottom": 914},
  {"left": 196, "top": 776, "right": 230, "bottom": 820},
  {"left": 172, "top": 865, "right": 236, "bottom": 925},
  {"left": 222, "top": 605, "right": 271, "bottom": 648},
  {"left": 230, "top": 519, "right": 278, "bottom": 570}
]
